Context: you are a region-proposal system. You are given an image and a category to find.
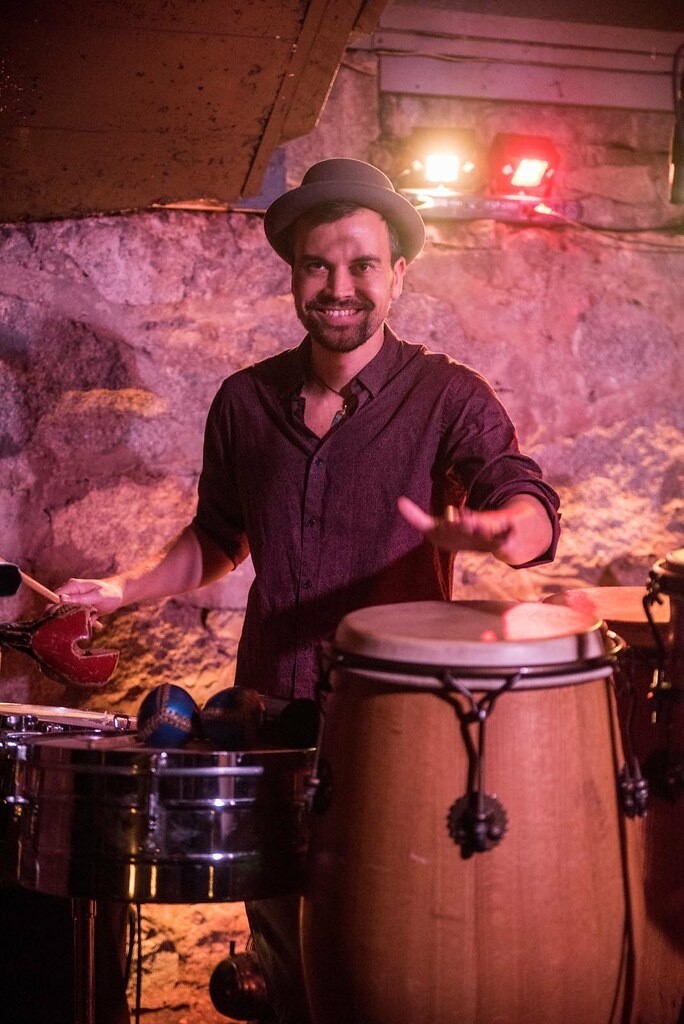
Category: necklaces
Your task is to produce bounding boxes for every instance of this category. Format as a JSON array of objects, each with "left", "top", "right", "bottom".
[{"left": 308, "top": 364, "right": 355, "bottom": 428}]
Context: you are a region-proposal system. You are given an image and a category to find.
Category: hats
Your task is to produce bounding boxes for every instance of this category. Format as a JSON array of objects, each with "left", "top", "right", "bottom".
[{"left": 265, "top": 159, "right": 427, "bottom": 267}]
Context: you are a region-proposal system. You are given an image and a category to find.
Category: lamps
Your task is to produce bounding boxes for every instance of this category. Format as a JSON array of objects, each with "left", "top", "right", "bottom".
[
  {"left": 394, "top": 126, "right": 475, "bottom": 188},
  {"left": 489, "top": 131, "right": 560, "bottom": 196}
]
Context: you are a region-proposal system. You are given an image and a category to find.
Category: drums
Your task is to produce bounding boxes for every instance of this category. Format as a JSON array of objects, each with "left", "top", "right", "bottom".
[
  {"left": 10, "top": 737, "right": 321, "bottom": 902},
  {"left": 299, "top": 600, "right": 650, "bottom": 1023},
  {"left": 0, "top": 700, "right": 141, "bottom": 737},
  {"left": 543, "top": 587, "right": 683, "bottom": 767}
]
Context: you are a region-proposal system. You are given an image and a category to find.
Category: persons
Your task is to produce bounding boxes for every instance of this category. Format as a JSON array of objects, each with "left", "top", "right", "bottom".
[{"left": 46, "top": 157, "right": 560, "bottom": 1024}]
